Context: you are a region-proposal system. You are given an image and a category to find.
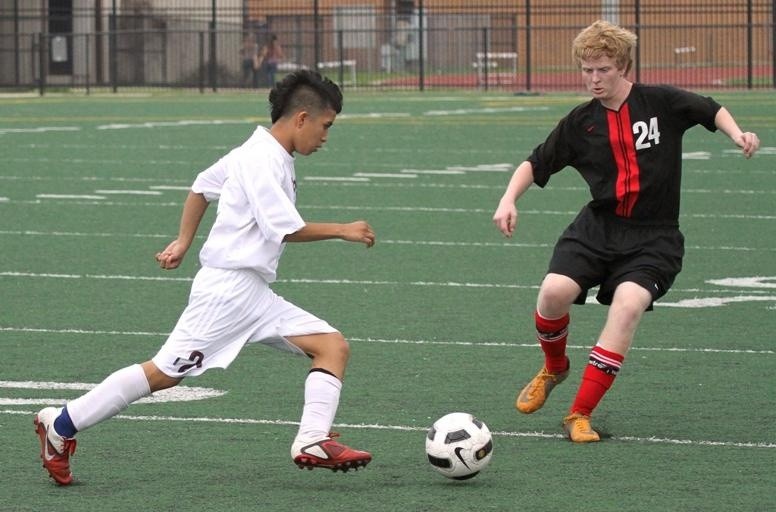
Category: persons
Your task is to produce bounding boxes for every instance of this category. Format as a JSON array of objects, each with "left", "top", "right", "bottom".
[
  {"left": 239, "top": 32, "right": 286, "bottom": 89},
  {"left": 492, "top": 19, "right": 762, "bottom": 443},
  {"left": 33, "top": 69, "right": 377, "bottom": 488}
]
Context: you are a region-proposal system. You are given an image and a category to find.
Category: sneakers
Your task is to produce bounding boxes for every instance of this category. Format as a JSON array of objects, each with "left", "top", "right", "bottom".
[
  {"left": 288, "top": 433, "right": 376, "bottom": 472},
  {"left": 560, "top": 409, "right": 602, "bottom": 444},
  {"left": 514, "top": 353, "right": 572, "bottom": 415},
  {"left": 32, "top": 402, "right": 77, "bottom": 486}
]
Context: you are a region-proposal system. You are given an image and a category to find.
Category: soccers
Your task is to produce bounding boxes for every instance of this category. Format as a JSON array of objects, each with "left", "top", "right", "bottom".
[{"left": 424, "top": 412, "right": 492, "bottom": 479}]
